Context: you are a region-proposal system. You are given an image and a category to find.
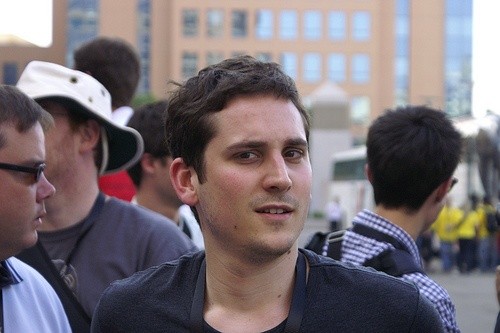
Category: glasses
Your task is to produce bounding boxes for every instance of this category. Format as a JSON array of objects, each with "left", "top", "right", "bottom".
[{"left": 0, "top": 160, "right": 46, "bottom": 183}]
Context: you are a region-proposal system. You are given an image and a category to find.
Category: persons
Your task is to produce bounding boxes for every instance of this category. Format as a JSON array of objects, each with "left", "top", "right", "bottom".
[
  {"left": 328, "top": 196, "right": 344, "bottom": 232},
  {"left": 16, "top": 61, "right": 198, "bottom": 333},
  {"left": 416, "top": 196, "right": 500, "bottom": 333},
  {"left": 90, "top": 55, "right": 446, "bottom": 333},
  {"left": 72, "top": 38, "right": 138, "bottom": 203},
  {"left": 0, "top": 85, "right": 74, "bottom": 333},
  {"left": 126, "top": 102, "right": 205, "bottom": 251},
  {"left": 303, "top": 105, "right": 460, "bottom": 333}
]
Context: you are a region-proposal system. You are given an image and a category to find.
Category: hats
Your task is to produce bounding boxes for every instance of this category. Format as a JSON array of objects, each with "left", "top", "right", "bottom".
[{"left": 16, "top": 60, "right": 144, "bottom": 174}]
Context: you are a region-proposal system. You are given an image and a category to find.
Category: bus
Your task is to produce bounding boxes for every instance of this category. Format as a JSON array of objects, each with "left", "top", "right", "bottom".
[{"left": 327, "top": 122, "right": 500, "bottom": 252}]
